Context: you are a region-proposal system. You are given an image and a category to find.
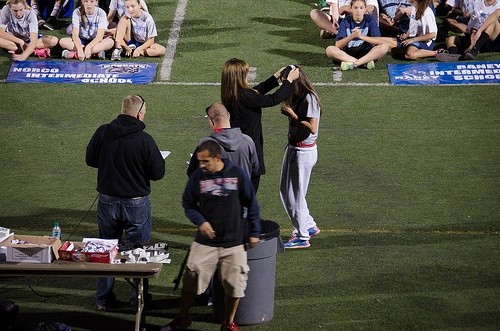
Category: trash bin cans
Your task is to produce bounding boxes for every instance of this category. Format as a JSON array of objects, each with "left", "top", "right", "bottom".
[{"left": 212, "top": 218, "right": 281, "bottom": 325}]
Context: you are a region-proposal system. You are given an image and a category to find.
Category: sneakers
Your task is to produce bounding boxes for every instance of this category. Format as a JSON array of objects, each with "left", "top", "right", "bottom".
[
  {"left": 128, "top": 44, "right": 136, "bottom": 49},
  {"left": 62, "top": 50, "right": 78, "bottom": 60},
  {"left": 436, "top": 53, "right": 461, "bottom": 62},
  {"left": 437, "top": 48, "right": 449, "bottom": 53},
  {"left": 161, "top": 314, "right": 192, "bottom": 331},
  {"left": 320, "top": 29, "right": 336, "bottom": 39},
  {"left": 284, "top": 236, "right": 310, "bottom": 248},
  {"left": 465, "top": 47, "right": 478, "bottom": 59},
  {"left": 34, "top": 48, "right": 50, "bottom": 58},
  {"left": 221, "top": 322, "right": 240, "bottom": 331},
  {"left": 364, "top": 61, "right": 375, "bottom": 69},
  {"left": 341, "top": 62, "right": 355, "bottom": 70},
  {"left": 91, "top": 51, "right": 105, "bottom": 60},
  {"left": 292, "top": 226, "right": 320, "bottom": 237},
  {"left": 111, "top": 49, "right": 122, "bottom": 60}
]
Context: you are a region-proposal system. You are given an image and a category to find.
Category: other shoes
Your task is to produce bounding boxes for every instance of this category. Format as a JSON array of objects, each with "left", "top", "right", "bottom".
[{"left": 98, "top": 304, "right": 110, "bottom": 310}]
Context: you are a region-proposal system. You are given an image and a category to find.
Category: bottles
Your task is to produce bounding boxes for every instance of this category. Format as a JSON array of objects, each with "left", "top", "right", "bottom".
[{"left": 52, "top": 222, "right": 61, "bottom": 240}]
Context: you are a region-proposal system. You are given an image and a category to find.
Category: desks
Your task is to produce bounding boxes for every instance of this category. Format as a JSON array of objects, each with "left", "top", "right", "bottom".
[{"left": 0, "top": 261, "right": 160, "bottom": 331}]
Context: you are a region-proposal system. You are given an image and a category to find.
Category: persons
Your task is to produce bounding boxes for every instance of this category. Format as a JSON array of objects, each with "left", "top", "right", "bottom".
[
  {"left": 310, "top": 0, "right": 500, "bottom": 70},
  {"left": 111, "top": 0, "right": 166, "bottom": 61},
  {"left": 279, "top": 65, "right": 322, "bottom": 248},
  {"left": 186, "top": 103, "right": 260, "bottom": 307},
  {"left": 86, "top": 95, "right": 165, "bottom": 312},
  {"left": 0, "top": 0, "right": 149, "bottom": 39},
  {"left": 221, "top": 58, "right": 299, "bottom": 175},
  {"left": 0, "top": 0, "right": 58, "bottom": 61},
  {"left": 59, "top": 0, "right": 114, "bottom": 61},
  {"left": 160, "top": 140, "right": 260, "bottom": 331}
]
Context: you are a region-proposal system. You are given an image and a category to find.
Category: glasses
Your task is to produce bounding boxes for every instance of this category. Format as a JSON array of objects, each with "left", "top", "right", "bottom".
[
  {"left": 137, "top": 95, "right": 145, "bottom": 112},
  {"left": 206, "top": 106, "right": 214, "bottom": 126}
]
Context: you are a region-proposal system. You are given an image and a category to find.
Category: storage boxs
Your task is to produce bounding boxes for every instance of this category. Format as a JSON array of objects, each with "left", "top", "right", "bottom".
[
  {"left": 0, "top": 235, "right": 62, "bottom": 263},
  {"left": 60, "top": 240, "right": 120, "bottom": 262}
]
